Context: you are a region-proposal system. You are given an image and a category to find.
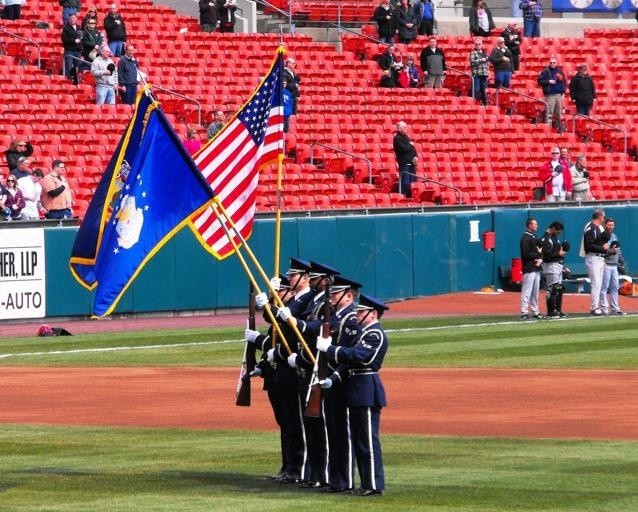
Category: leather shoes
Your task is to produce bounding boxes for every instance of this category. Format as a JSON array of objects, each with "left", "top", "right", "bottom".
[
  {"left": 269, "top": 471, "right": 301, "bottom": 485},
  {"left": 322, "top": 483, "right": 356, "bottom": 495},
  {"left": 297, "top": 478, "right": 328, "bottom": 489},
  {"left": 343, "top": 487, "right": 384, "bottom": 499}
]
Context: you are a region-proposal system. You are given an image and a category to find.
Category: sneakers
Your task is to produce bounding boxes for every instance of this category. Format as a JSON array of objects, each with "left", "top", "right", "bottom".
[{"left": 520, "top": 307, "right": 627, "bottom": 320}]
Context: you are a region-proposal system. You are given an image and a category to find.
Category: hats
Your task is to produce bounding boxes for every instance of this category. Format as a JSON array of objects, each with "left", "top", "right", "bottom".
[
  {"left": 285, "top": 257, "right": 311, "bottom": 276},
  {"left": 97, "top": 42, "right": 110, "bottom": 56},
  {"left": 305, "top": 261, "right": 342, "bottom": 279},
  {"left": 325, "top": 275, "right": 362, "bottom": 294},
  {"left": 276, "top": 272, "right": 291, "bottom": 291},
  {"left": 352, "top": 294, "right": 391, "bottom": 314}
]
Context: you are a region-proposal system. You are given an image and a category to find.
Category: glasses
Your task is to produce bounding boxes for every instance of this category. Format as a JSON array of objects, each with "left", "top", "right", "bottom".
[
  {"left": 551, "top": 152, "right": 559, "bottom": 155},
  {"left": 550, "top": 61, "right": 556, "bottom": 64},
  {"left": 474, "top": 40, "right": 507, "bottom": 45},
  {"left": 7, "top": 179, "right": 15, "bottom": 183},
  {"left": 17, "top": 144, "right": 27, "bottom": 147},
  {"left": 87, "top": 10, "right": 97, "bottom": 24}
]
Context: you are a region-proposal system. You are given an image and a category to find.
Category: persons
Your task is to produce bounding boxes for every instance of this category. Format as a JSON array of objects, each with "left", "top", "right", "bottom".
[
  {"left": 559, "top": 148, "right": 573, "bottom": 168},
  {"left": 567, "top": 64, "right": 597, "bottom": 116},
  {"left": 243, "top": 257, "right": 390, "bottom": 497},
  {"left": 0, "top": 139, "right": 74, "bottom": 221},
  {"left": 283, "top": 76, "right": 295, "bottom": 134},
  {"left": 540, "top": 220, "right": 570, "bottom": 318},
  {"left": 394, "top": 119, "right": 417, "bottom": 197},
  {"left": 199, "top": 1, "right": 220, "bottom": 33},
  {"left": 182, "top": 126, "right": 203, "bottom": 155},
  {"left": 537, "top": 147, "right": 573, "bottom": 202},
  {"left": 360, "top": 0, "right": 544, "bottom": 106},
  {"left": 537, "top": 58, "right": 567, "bottom": 134},
  {"left": 58, "top": 2, "right": 139, "bottom": 108},
  {"left": 602, "top": 218, "right": 625, "bottom": 316},
  {"left": 1, "top": 0, "right": 29, "bottom": 20},
  {"left": 209, "top": 109, "right": 225, "bottom": 137},
  {"left": 569, "top": 154, "right": 592, "bottom": 204},
  {"left": 284, "top": 57, "right": 301, "bottom": 116},
  {"left": 582, "top": 208, "right": 611, "bottom": 316},
  {"left": 218, "top": 0, "right": 238, "bottom": 33},
  {"left": 519, "top": 218, "right": 544, "bottom": 319}
]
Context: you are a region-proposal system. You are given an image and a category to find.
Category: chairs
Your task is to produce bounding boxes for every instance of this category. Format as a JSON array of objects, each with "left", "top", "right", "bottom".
[{"left": 0, "top": 0, "right": 638, "bottom": 226}]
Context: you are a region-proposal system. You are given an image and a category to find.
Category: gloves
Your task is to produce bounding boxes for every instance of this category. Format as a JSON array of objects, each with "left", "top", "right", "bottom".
[
  {"left": 287, "top": 351, "right": 299, "bottom": 369},
  {"left": 278, "top": 306, "right": 291, "bottom": 321},
  {"left": 268, "top": 276, "right": 282, "bottom": 292},
  {"left": 266, "top": 348, "right": 277, "bottom": 363},
  {"left": 319, "top": 377, "right": 333, "bottom": 390},
  {"left": 250, "top": 364, "right": 263, "bottom": 377},
  {"left": 255, "top": 291, "right": 269, "bottom": 309},
  {"left": 243, "top": 329, "right": 261, "bottom": 344},
  {"left": 314, "top": 335, "right": 333, "bottom": 352}
]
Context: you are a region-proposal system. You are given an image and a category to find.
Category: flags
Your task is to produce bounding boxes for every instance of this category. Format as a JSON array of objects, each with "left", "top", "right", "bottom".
[
  {"left": 69, "top": 83, "right": 221, "bottom": 320},
  {"left": 176, "top": 40, "right": 284, "bottom": 259}
]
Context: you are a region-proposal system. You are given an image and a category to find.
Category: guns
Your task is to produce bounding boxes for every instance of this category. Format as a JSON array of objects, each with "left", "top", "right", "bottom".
[
  {"left": 305, "top": 281, "right": 329, "bottom": 417},
  {"left": 234, "top": 281, "right": 256, "bottom": 407}
]
